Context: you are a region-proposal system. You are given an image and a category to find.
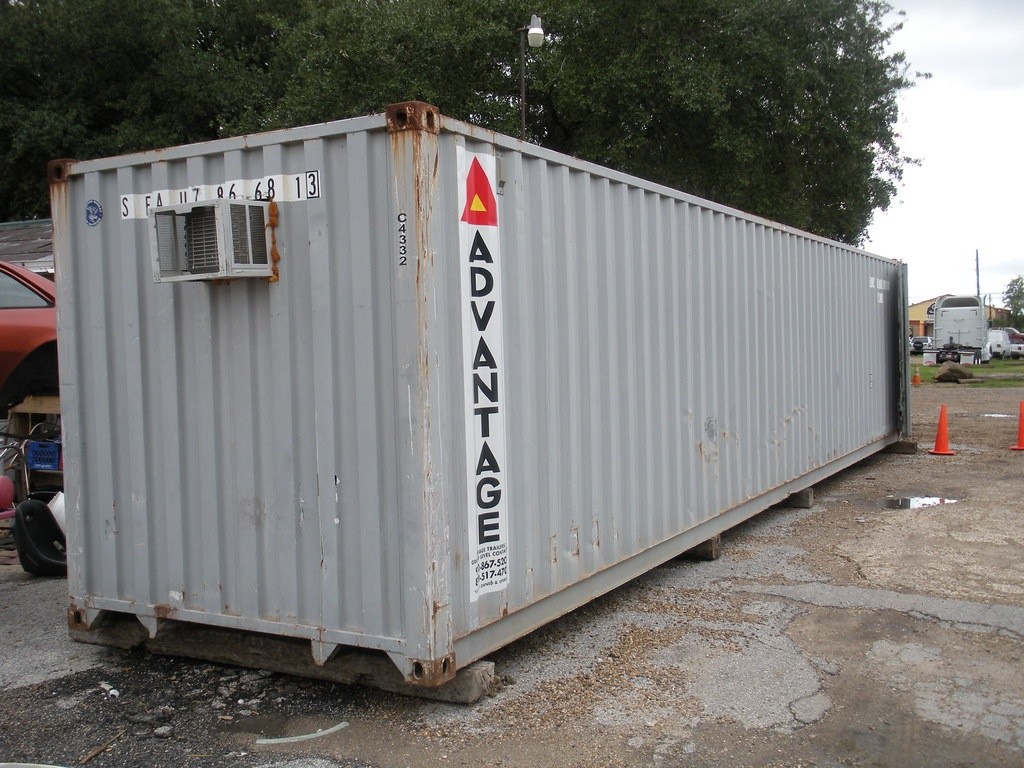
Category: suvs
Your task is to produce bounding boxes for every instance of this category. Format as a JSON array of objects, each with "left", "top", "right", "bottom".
[
  {"left": 909, "top": 336, "right": 933, "bottom": 356},
  {"left": 989, "top": 327, "right": 1024, "bottom": 344}
]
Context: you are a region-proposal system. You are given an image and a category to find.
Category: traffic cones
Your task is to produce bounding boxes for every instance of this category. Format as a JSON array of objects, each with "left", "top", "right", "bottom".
[
  {"left": 911, "top": 362, "right": 924, "bottom": 385},
  {"left": 927, "top": 404, "right": 954, "bottom": 455},
  {"left": 1008, "top": 400, "right": 1024, "bottom": 450}
]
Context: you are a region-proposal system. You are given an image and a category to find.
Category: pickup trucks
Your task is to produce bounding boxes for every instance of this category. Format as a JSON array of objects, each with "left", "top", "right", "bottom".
[{"left": 1011, "top": 344, "right": 1024, "bottom": 360}]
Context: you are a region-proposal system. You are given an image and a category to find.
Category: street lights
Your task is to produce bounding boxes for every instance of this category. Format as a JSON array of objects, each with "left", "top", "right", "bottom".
[{"left": 515, "top": 13, "right": 545, "bottom": 141}]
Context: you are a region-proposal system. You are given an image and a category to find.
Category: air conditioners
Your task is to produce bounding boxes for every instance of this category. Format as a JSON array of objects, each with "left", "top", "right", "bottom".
[{"left": 149, "top": 198, "right": 275, "bottom": 283}]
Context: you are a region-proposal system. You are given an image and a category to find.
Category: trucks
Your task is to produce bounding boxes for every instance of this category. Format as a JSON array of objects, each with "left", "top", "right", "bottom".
[{"left": 932, "top": 294, "right": 988, "bottom": 365}]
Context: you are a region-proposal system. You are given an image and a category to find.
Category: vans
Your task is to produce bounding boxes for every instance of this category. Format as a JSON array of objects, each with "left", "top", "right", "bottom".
[{"left": 988, "top": 329, "right": 1012, "bottom": 361}]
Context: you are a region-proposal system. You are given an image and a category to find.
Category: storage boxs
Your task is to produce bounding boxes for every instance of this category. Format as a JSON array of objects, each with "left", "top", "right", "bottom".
[
  {"left": 45, "top": 102, "right": 913, "bottom": 687},
  {"left": 25, "top": 439, "right": 60, "bottom": 470}
]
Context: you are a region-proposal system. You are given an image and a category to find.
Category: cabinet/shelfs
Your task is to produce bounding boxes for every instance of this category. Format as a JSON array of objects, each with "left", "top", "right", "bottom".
[{"left": 12, "top": 397, "right": 63, "bottom": 499}]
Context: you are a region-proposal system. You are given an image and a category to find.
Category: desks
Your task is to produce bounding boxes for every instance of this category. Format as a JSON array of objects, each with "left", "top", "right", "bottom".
[{"left": 910, "top": 349, "right": 981, "bottom": 364}]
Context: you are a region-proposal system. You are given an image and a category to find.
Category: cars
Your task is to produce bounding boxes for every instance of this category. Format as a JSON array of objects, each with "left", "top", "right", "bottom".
[{"left": 0, "top": 262, "right": 60, "bottom": 411}]
